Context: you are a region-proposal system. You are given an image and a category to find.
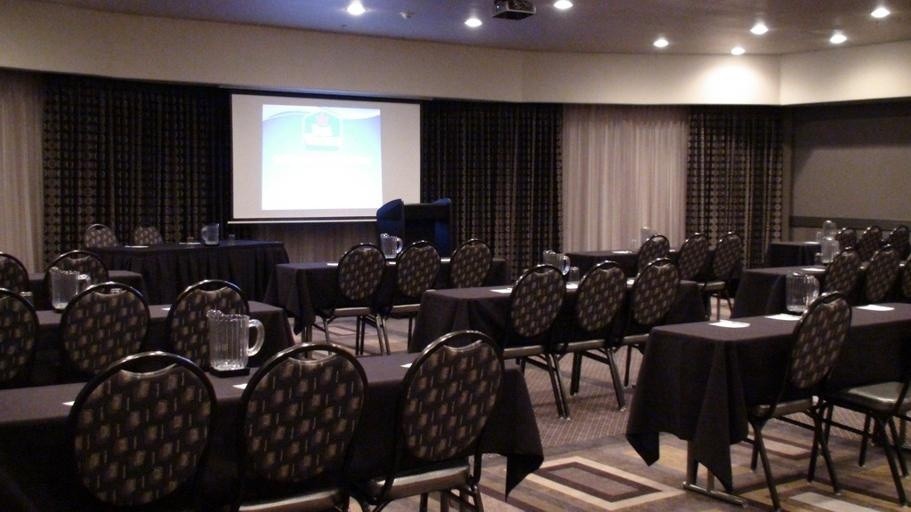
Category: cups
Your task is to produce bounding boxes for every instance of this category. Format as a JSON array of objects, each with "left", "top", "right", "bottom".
[
  {"left": 568, "top": 267, "right": 579, "bottom": 284},
  {"left": 228, "top": 234, "right": 235, "bottom": 246},
  {"left": 48, "top": 266, "right": 92, "bottom": 309},
  {"left": 821, "top": 237, "right": 840, "bottom": 265},
  {"left": 200, "top": 223, "right": 220, "bottom": 245},
  {"left": 815, "top": 253, "right": 822, "bottom": 265},
  {"left": 543, "top": 250, "right": 571, "bottom": 276},
  {"left": 380, "top": 234, "right": 403, "bottom": 259},
  {"left": 784, "top": 272, "right": 820, "bottom": 315},
  {"left": 206, "top": 309, "right": 266, "bottom": 371}
]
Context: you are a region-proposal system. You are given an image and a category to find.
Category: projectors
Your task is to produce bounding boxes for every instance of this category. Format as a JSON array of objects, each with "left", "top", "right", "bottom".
[{"left": 492, "top": 0, "right": 536, "bottom": 20}]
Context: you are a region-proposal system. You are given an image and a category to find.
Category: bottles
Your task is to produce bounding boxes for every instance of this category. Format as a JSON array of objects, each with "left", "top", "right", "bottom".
[{"left": 20, "top": 291, "right": 33, "bottom": 303}]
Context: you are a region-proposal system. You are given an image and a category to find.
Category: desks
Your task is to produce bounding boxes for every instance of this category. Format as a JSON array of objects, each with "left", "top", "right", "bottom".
[{"left": 1, "top": 347, "right": 521, "bottom": 511}]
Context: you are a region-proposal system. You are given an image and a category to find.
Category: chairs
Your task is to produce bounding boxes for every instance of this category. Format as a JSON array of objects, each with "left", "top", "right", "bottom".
[
  {"left": 57, "top": 281, "right": 152, "bottom": 381},
  {"left": 574, "top": 257, "right": 682, "bottom": 387},
  {"left": 0, "top": 286, "right": 40, "bottom": 388},
  {"left": 83, "top": 222, "right": 288, "bottom": 300},
  {"left": 2, "top": 250, "right": 285, "bottom": 352},
  {"left": 273, "top": 219, "right": 909, "bottom": 361},
  {"left": 649, "top": 303, "right": 910, "bottom": 511},
  {"left": 58, "top": 351, "right": 217, "bottom": 509},
  {"left": 504, "top": 264, "right": 565, "bottom": 420},
  {"left": 165, "top": 280, "right": 250, "bottom": 371},
  {"left": 518, "top": 260, "right": 624, "bottom": 422},
  {"left": 353, "top": 327, "right": 507, "bottom": 512},
  {"left": 223, "top": 340, "right": 368, "bottom": 511}
]
